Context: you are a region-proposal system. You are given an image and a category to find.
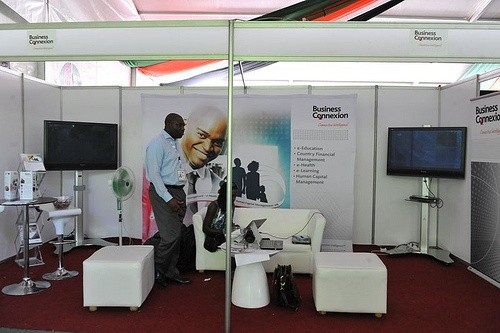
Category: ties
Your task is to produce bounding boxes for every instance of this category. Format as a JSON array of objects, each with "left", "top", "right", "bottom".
[{"left": 186, "top": 172, "right": 198, "bottom": 214}]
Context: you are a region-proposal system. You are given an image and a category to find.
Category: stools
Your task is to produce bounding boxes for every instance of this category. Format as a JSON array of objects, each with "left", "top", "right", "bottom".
[
  {"left": 83, "top": 245, "right": 154, "bottom": 311},
  {"left": 312, "top": 252, "right": 387, "bottom": 317},
  {"left": 42, "top": 207, "right": 81, "bottom": 281}
]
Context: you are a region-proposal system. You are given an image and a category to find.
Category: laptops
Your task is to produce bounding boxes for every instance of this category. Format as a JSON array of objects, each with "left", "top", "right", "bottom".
[
  {"left": 241, "top": 219, "right": 267, "bottom": 233},
  {"left": 249, "top": 222, "right": 283, "bottom": 250}
]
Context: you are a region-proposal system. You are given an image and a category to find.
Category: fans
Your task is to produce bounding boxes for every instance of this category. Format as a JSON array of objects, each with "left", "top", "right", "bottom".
[{"left": 112, "top": 166, "right": 135, "bottom": 246}]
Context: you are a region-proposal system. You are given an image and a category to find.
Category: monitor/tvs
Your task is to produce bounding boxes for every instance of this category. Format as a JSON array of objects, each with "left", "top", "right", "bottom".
[
  {"left": 44, "top": 120, "right": 118, "bottom": 169},
  {"left": 387, "top": 127, "right": 466, "bottom": 178}
]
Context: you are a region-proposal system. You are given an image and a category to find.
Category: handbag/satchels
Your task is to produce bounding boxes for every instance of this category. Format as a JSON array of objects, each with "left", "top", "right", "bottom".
[{"left": 271, "top": 264, "right": 300, "bottom": 311}]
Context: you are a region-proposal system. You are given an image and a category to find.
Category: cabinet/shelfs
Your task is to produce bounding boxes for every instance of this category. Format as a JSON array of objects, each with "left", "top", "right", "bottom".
[{"left": 15, "top": 206, "right": 45, "bottom": 268}]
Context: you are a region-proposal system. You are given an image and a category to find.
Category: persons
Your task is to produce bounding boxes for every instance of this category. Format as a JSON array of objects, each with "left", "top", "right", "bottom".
[
  {"left": 144, "top": 112, "right": 192, "bottom": 286},
  {"left": 182, "top": 108, "right": 227, "bottom": 169},
  {"left": 203, "top": 181, "right": 240, "bottom": 282}
]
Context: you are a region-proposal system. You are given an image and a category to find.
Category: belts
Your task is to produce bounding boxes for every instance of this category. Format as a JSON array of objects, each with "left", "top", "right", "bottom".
[{"left": 166, "top": 185, "right": 184, "bottom": 189}]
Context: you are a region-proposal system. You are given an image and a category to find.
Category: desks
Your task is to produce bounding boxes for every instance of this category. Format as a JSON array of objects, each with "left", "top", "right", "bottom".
[
  {"left": 221, "top": 241, "right": 280, "bottom": 308},
  {"left": 0, "top": 197, "right": 58, "bottom": 295}
]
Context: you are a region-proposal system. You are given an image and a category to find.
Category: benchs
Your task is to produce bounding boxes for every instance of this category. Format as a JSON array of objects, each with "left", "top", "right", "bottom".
[{"left": 192, "top": 207, "right": 327, "bottom": 274}]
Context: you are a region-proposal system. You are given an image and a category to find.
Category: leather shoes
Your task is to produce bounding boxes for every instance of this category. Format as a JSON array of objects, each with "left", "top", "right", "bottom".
[
  {"left": 154, "top": 272, "right": 168, "bottom": 288},
  {"left": 174, "top": 278, "right": 192, "bottom": 284}
]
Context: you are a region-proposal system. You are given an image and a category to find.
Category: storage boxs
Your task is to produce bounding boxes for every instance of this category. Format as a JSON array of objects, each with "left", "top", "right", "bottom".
[{"left": 4, "top": 171, "right": 18, "bottom": 201}]
